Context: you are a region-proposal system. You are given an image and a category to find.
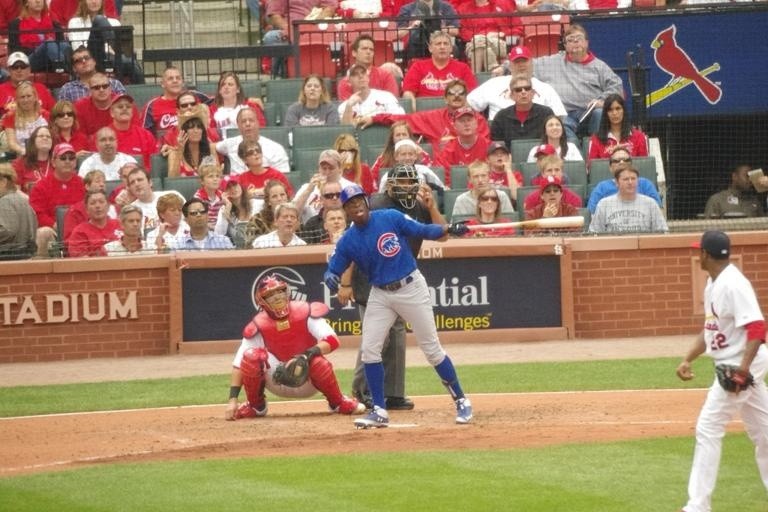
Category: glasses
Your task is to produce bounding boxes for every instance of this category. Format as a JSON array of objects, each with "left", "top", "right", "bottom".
[
  {"left": 544, "top": 187, "right": 560, "bottom": 192},
  {"left": 75, "top": 55, "right": 92, "bottom": 63},
  {"left": 190, "top": 209, "right": 206, "bottom": 215},
  {"left": 54, "top": 155, "right": 75, "bottom": 161},
  {"left": 181, "top": 102, "right": 196, "bottom": 108},
  {"left": 446, "top": 89, "right": 465, "bottom": 96},
  {"left": 480, "top": 196, "right": 498, "bottom": 202},
  {"left": 246, "top": 148, "right": 261, "bottom": 156},
  {"left": 90, "top": 83, "right": 109, "bottom": 89},
  {"left": 13, "top": 64, "right": 27, "bottom": 69},
  {"left": 611, "top": 157, "right": 630, "bottom": 163},
  {"left": 56, "top": 112, "right": 73, "bottom": 117},
  {"left": 513, "top": 85, "right": 532, "bottom": 92},
  {"left": 322, "top": 191, "right": 341, "bottom": 199}
]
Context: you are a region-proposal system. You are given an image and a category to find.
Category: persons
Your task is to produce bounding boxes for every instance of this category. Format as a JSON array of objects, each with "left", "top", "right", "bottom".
[
  {"left": 258, "top": 0, "right": 671, "bottom": 243},
  {"left": 1, "top": 0, "right": 147, "bottom": 84},
  {"left": 334, "top": 162, "right": 451, "bottom": 411},
  {"left": 226, "top": 273, "right": 366, "bottom": 420},
  {"left": 705, "top": 162, "right": 768, "bottom": 219},
  {"left": 674, "top": 231, "right": 767, "bottom": 512},
  {"left": 1, "top": 47, "right": 310, "bottom": 262},
  {"left": 322, "top": 181, "right": 476, "bottom": 427}
]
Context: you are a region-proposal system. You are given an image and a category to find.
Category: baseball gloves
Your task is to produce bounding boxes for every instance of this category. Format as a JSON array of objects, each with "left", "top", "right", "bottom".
[
  {"left": 272, "top": 354, "right": 309, "bottom": 387},
  {"left": 716, "top": 364, "right": 753, "bottom": 392}
]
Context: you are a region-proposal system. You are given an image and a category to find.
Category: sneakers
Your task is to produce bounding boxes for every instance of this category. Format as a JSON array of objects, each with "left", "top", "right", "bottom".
[
  {"left": 454, "top": 398, "right": 472, "bottom": 423},
  {"left": 328, "top": 390, "right": 413, "bottom": 427},
  {"left": 235, "top": 402, "right": 267, "bottom": 417}
]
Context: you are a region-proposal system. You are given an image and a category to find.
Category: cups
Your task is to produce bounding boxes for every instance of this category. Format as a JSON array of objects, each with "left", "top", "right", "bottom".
[
  {"left": 331, "top": 43, "right": 341, "bottom": 62},
  {"left": 392, "top": 40, "right": 405, "bottom": 64},
  {"left": 505, "top": 35, "right": 517, "bottom": 55}
]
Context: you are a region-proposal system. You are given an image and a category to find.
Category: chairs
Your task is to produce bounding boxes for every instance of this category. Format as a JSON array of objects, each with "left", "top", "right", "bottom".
[{"left": 48, "top": 15, "right": 661, "bottom": 258}]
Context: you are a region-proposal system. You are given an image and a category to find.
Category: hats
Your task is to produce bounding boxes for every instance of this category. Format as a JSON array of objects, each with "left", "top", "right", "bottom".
[
  {"left": 52, "top": 143, "right": 76, "bottom": 157},
  {"left": 488, "top": 141, "right": 507, "bottom": 155},
  {"left": 541, "top": 176, "right": 560, "bottom": 194},
  {"left": 692, "top": 232, "right": 731, "bottom": 257},
  {"left": 347, "top": 64, "right": 368, "bottom": 78},
  {"left": 319, "top": 150, "right": 340, "bottom": 168},
  {"left": 111, "top": 91, "right": 133, "bottom": 104},
  {"left": 7, "top": 52, "right": 30, "bottom": 67},
  {"left": 534, "top": 144, "right": 556, "bottom": 157},
  {"left": 178, "top": 110, "right": 208, "bottom": 131},
  {"left": 508, "top": 46, "right": 531, "bottom": 62},
  {"left": 220, "top": 175, "right": 240, "bottom": 191}
]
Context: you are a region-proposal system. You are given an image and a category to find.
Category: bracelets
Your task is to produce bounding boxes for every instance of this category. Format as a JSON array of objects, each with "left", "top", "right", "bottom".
[
  {"left": 306, "top": 345, "right": 322, "bottom": 358},
  {"left": 229, "top": 386, "right": 241, "bottom": 399},
  {"left": 340, "top": 283, "right": 354, "bottom": 289}
]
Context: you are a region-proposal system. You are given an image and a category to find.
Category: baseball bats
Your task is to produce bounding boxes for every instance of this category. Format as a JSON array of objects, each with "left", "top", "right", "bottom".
[{"left": 466, "top": 217, "right": 584, "bottom": 231}]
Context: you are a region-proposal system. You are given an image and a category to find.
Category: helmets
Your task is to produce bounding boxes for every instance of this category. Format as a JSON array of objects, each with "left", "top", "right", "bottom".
[
  {"left": 388, "top": 164, "right": 419, "bottom": 210},
  {"left": 340, "top": 186, "right": 366, "bottom": 205},
  {"left": 256, "top": 276, "right": 289, "bottom": 319}
]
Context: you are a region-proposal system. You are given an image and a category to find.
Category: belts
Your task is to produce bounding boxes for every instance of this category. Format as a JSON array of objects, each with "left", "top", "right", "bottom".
[{"left": 380, "top": 277, "right": 412, "bottom": 291}]
